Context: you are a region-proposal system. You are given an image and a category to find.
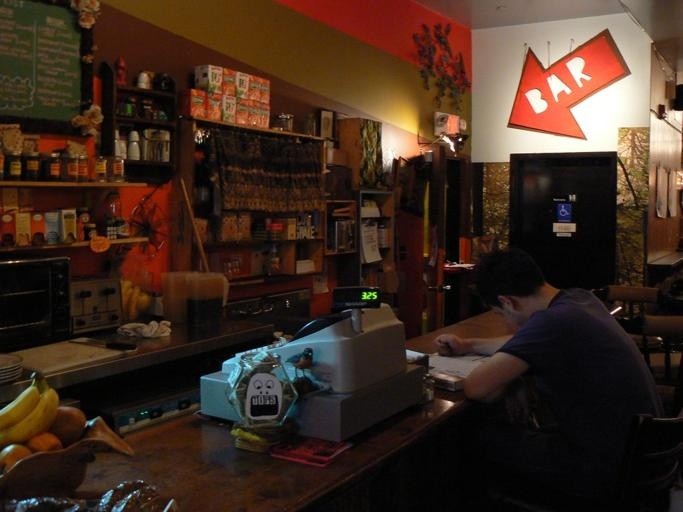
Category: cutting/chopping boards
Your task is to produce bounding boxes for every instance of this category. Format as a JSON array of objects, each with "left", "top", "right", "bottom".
[{"left": 7, "top": 339, "right": 139, "bottom": 379}]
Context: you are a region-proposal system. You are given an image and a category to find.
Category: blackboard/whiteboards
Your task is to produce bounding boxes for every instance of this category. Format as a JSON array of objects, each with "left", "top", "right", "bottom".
[{"left": 0, "top": 1, "right": 96, "bottom": 135}]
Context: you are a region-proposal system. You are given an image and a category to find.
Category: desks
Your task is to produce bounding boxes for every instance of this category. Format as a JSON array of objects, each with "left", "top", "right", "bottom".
[{"left": 70, "top": 312, "right": 525, "bottom": 511}]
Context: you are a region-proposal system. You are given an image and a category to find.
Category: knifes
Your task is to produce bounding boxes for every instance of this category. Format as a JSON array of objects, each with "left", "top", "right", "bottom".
[{"left": 71, "top": 335, "right": 136, "bottom": 351}]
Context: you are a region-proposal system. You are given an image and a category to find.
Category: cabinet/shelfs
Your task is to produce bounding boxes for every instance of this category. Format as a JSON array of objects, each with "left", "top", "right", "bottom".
[
  {"left": 324, "top": 188, "right": 397, "bottom": 308},
  {"left": 0, "top": 178, "right": 149, "bottom": 253},
  {"left": 101, "top": 62, "right": 177, "bottom": 189},
  {"left": 172, "top": 117, "right": 325, "bottom": 288}
]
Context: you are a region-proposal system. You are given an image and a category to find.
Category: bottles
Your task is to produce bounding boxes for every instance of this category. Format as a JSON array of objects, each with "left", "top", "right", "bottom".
[
  {"left": 78, "top": 191, "right": 130, "bottom": 241},
  {"left": 129, "top": 131, "right": 140, "bottom": 161},
  {"left": 0, "top": 149, "right": 125, "bottom": 183},
  {"left": 226, "top": 353, "right": 298, "bottom": 449}
]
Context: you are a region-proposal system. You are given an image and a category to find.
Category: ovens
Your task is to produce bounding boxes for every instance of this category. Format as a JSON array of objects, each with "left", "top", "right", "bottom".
[{"left": 0, "top": 255, "right": 71, "bottom": 353}]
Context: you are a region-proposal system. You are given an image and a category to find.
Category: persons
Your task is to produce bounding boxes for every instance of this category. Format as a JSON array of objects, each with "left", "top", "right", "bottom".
[{"left": 433, "top": 250, "right": 655, "bottom": 508}]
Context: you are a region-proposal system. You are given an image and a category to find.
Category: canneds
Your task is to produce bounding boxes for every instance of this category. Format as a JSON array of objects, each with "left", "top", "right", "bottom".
[
  {"left": 82, "top": 223, "right": 97, "bottom": 240},
  {"left": 77, "top": 206, "right": 91, "bottom": 223},
  {"left": 3, "top": 152, "right": 125, "bottom": 183}
]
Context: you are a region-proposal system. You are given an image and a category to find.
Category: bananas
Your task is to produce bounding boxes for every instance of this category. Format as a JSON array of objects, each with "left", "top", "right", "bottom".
[{"left": 0, "top": 367, "right": 59, "bottom": 450}]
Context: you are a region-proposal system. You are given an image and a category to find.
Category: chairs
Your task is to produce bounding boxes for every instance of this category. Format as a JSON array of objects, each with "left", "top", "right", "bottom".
[
  {"left": 461, "top": 409, "right": 682, "bottom": 509},
  {"left": 594, "top": 283, "right": 682, "bottom": 404}
]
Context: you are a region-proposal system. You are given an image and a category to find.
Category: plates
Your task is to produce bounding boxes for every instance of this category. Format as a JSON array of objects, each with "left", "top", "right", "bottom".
[{"left": 0, "top": 354, "right": 24, "bottom": 387}]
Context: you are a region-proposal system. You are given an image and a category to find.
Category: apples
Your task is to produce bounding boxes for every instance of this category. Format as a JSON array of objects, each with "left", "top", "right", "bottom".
[
  {"left": 49, "top": 405, "right": 86, "bottom": 442},
  {"left": 24, "top": 431, "right": 64, "bottom": 454},
  {"left": 1, "top": 443, "right": 32, "bottom": 475}
]
